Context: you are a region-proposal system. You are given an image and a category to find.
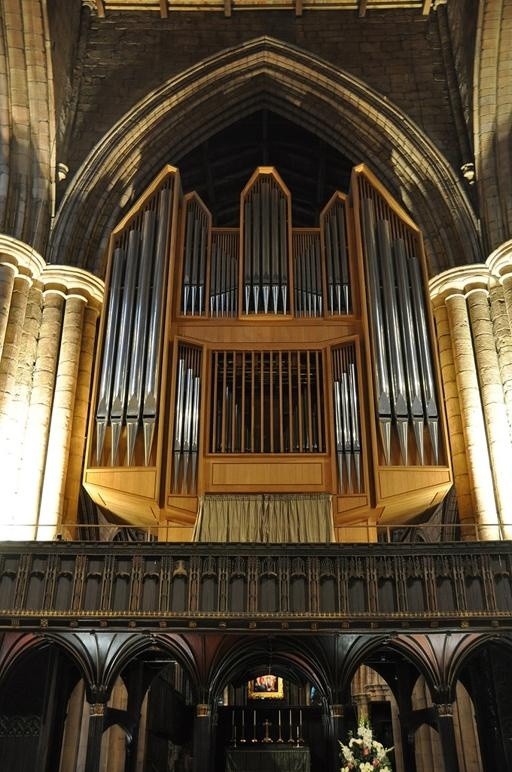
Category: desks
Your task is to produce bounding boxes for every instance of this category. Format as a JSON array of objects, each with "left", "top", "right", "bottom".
[{"left": 225, "top": 746, "right": 310, "bottom": 772}]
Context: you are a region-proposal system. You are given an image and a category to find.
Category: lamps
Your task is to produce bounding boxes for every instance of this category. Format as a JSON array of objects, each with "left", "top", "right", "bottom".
[{"left": 197, "top": 694, "right": 208, "bottom": 718}]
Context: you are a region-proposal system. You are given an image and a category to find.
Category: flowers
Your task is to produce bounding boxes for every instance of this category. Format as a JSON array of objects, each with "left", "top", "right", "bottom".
[{"left": 337, "top": 709, "right": 395, "bottom": 771}]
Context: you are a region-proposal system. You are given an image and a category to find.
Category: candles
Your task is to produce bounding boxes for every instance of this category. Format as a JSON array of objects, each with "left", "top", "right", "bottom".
[
  {"left": 297, "top": 726, "right": 299, "bottom": 738},
  {"left": 242, "top": 709, "right": 244, "bottom": 727},
  {"left": 279, "top": 710, "right": 281, "bottom": 728},
  {"left": 235, "top": 726, "right": 236, "bottom": 737},
  {"left": 289, "top": 709, "right": 292, "bottom": 728},
  {"left": 300, "top": 709, "right": 302, "bottom": 727},
  {"left": 254, "top": 709, "right": 256, "bottom": 727},
  {"left": 232, "top": 710, "right": 234, "bottom": 725}
]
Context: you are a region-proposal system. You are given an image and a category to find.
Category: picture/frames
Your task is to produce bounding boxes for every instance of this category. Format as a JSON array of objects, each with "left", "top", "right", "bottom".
[{"left": 248, "top": 675, "right": 284, "bottom": 699}]
